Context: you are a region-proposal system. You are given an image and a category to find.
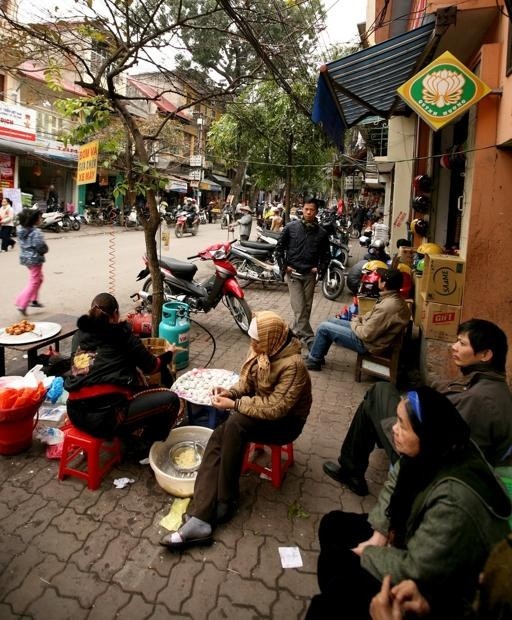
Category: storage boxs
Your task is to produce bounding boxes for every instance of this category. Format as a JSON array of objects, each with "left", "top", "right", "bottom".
[
  {"left": 139, "top": 337, "right": 175, "bottom": 385},
  {"left": 420, "top": 300, "right": 461, "bottom": 342},
  {"left": 358, "top": 295, "right": 414, "bottom": 318},
  {"left": 413, "top": 272, "right": 423, "bottom": 325},
  {"left": 420, "top": 254, "right": 465, "bottom": 305}
]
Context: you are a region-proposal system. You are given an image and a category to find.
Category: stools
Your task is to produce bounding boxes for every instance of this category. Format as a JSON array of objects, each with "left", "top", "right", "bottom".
[
  {"left": 241, "top": 442, "right": 293, "bottom": 489},
  {"left": 58, "top": 426, "right": 121, "bottom": 491},
  {"left": 187, "top": 401, "right": 226, "bottom": 428}
]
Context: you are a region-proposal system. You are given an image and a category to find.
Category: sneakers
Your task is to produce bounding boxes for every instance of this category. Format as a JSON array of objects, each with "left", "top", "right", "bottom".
[
  {"left": 305, "top": 358, "right": 321, "bottom": 371},
  {"left": 308, "top": 341, "right": 313, "bottom": 351}
]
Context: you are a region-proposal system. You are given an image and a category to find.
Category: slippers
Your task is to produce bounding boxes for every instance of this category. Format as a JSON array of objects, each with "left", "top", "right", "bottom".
[{"left": 158, "top": 532, "right": 213, "bottom": 547}]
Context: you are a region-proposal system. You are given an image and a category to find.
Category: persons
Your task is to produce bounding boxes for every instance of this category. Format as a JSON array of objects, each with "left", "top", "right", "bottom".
[
  {"left": 229, "top": 199, "right": 285, "bottom": 243},
  {"left": 274, "top": 199, "right": 331, "bottom": 348},
  {"left": 1, "top": 198, "right": 16, "bottom": 251},
  {"left": 370, "top": 576, "right": 440, "bottom": 620},
  {"left": 14, "top": 209, "right": 48, "bottom": 315},
  {"left": 46, "top": 185, "right": 76, "bottom": 216},
  {"left": 348, "top": 203, "right": 371, "bottom": 246},
  {"left": 64, "top": 293, "right": 180, "bottom": 468},
  {"left": 157, "top": 311, "right": 311, "bottom": 552},
  {"left": 298, "top": 394, "right": 511, "bottom": 620},
  {"left": 303, "top": 238, "right": 411, "bottom": 371},
  {"left": 173, "top": 196, "right": 218, "bottom": 228},
  {"left": 322, "top": 318, "right": 512, "bottom": 499}
]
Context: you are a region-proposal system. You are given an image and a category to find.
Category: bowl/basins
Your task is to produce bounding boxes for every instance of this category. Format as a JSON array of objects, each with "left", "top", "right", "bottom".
[
  {"left": 169, "top": 440, "right": 205, "bottom": 473},
  {"left": 148, "top": 426, "right": 214, "bottom": 498}
]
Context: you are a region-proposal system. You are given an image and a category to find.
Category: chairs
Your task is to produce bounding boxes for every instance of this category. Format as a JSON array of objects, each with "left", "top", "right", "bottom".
[{"left": 353, "top": 320, "right": 413, "bottom": 381}]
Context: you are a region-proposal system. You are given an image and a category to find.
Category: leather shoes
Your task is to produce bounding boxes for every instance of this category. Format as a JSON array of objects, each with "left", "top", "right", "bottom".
[{"left": 323, "top": 461, "right": 368, "bottom": 496}]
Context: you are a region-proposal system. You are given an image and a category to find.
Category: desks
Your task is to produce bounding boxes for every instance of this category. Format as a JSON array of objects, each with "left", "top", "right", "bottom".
[{"left": 0, "top": 313, "right": 79, "bottom": 375}]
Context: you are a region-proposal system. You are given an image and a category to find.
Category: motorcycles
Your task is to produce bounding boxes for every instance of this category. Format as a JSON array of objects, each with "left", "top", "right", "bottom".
[
  {"left": 220, "top": 206, "right": 234, "bottom": 229},
  {"left": 198, "top": 208, "right": 206, "bottom": 223},
  {"left": 137, "top": 239, "right": 251, "bottom": 335},
  {"left": 175, "top": 215, "right": 200, "bottom": 238},
  {"left": 317, "top": 204, "right": 361, "bottom": 238},
  {"left": 229, "top": 225, "right": 351, "bottom": 299},
  {"left": 27, "top": 203, "right": 107, "bottom": 233}
]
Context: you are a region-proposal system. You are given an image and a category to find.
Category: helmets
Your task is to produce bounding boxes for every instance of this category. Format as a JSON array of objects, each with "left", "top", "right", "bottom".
[
  {"left": 359, "top": 260, "right": 389, "bottom": 292},
  {"left": 411, "top": 175, "right": 444, "bottom": 272}
]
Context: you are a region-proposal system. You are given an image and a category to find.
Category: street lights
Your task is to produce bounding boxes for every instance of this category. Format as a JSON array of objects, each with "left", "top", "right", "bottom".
[{"left": 194, "top": 113, "right": 207, "bottom": 184}]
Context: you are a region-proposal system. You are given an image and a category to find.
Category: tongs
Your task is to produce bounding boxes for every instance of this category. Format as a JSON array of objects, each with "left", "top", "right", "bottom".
[{"left": 31, "top": 328, "right": 42, "bottom": 336}]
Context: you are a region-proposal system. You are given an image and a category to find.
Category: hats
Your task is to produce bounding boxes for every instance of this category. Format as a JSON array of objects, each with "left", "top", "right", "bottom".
[
  {"left": 377, "top": 268, "right": 403, "bottom": 288},
  {"left": 248, "top": 317, "right": 259, "bottom": 341},
  {"left": 240, "top": 206, "right": 253, "bottom": 214}
]
